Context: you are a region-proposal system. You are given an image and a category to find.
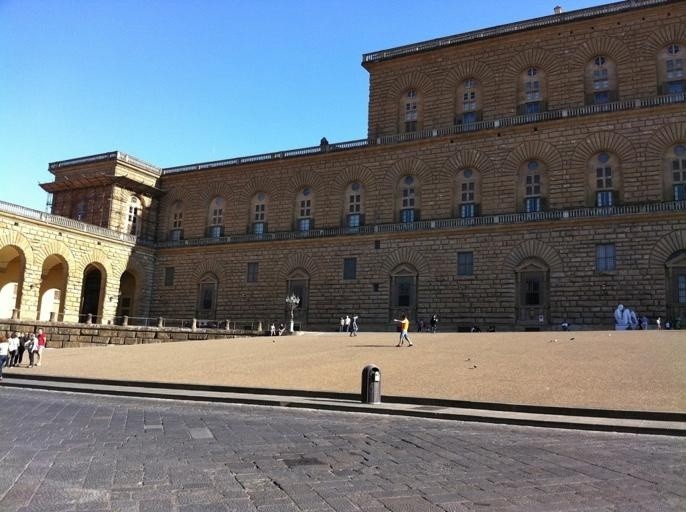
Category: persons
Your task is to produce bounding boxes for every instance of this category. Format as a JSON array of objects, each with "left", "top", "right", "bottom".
[
  {"left": 340, "top": 317, "right": 345, "bottom": 332},
  {"left": 614, "top": 304, "right": 637, "bottom": 325},
  {"left": 393, "top": 314, "right": 413, "bottom": 347},
  {"left": 270, "top": 323, "right": 276, "bottom": 336},
  {"left": 0, "top": 327, "right": 46, "bottom": 380},
  {"left": 345, "top": 316, "right": 351, "bottom": 332},
  {"left": 637, "top": 315, "right": 662, "bottom": 332},
  {"left": 350, "top": 315, "right": 358, "bottom": 337},
  {"left": 560, "top": 322, "right": 570, "bottom": 330},
  {"left": 417, "top": 311, "right": 439, "bottom": 333},
  {"left": 278, "top": 324, "right": 285, "bottom": 336}
]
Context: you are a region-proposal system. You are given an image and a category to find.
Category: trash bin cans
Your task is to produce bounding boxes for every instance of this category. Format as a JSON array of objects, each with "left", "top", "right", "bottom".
[{"left": 362, "top": 364, "right": 381, "bottom": 405}]
